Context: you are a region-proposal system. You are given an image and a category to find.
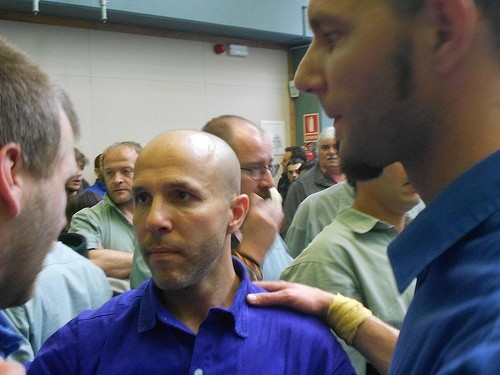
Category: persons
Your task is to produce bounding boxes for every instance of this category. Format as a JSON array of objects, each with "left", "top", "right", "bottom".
[
  {"left": 2, "top": 115, "right": 427, "bottom": 375},
  {"left": 0, "top": 37, "right": 81, "bottom": 375},
  {"left": 294, "top": 0, "right": 500, "bottom": 375}
]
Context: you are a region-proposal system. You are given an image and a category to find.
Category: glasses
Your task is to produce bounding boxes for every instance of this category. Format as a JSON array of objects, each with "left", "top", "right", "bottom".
[{"left": 240, "top": 164, "right": 281, "bottom": 181}]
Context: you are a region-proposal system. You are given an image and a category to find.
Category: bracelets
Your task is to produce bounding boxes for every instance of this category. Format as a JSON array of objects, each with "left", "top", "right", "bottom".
[{"left": 238, "top": 252, "right": 260, "bottom": 267}]
